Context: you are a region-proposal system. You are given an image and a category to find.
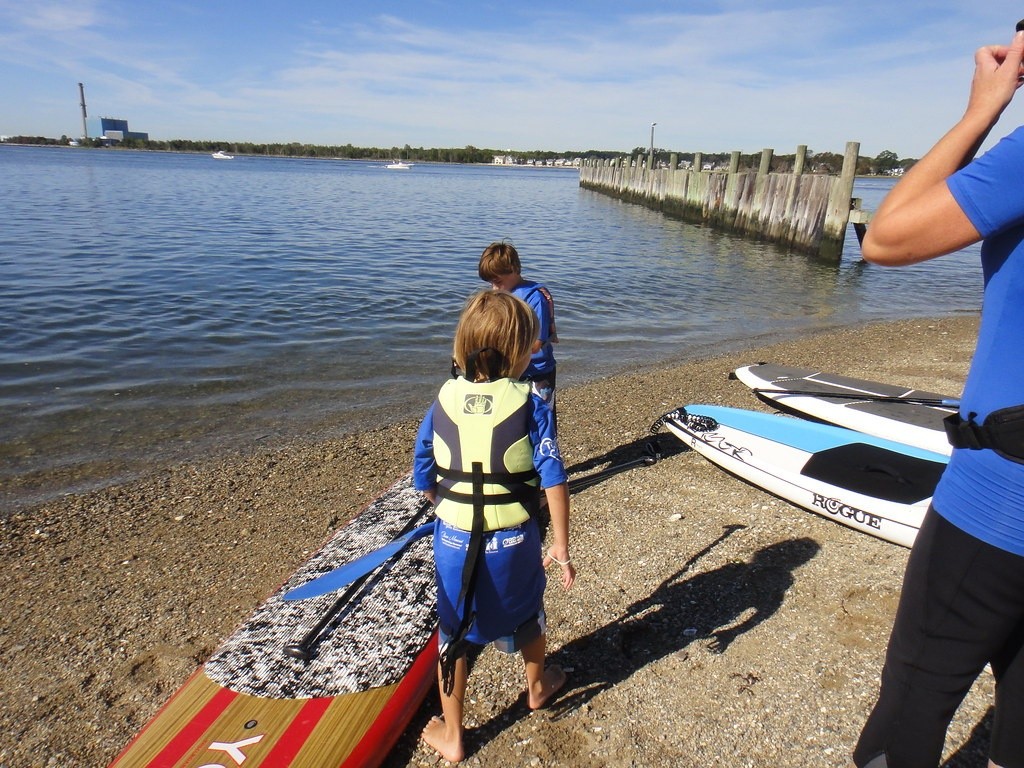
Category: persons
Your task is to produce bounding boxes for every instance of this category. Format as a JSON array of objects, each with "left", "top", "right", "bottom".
[
  {"left": 479, "top": 242, "right": 560, "bottom": 446},
  {"left": 413, "top": 289, "right": 578, "bottom": 763},
  {"left": 851, "top": 25, "right": 1024, "bottom": 768}
]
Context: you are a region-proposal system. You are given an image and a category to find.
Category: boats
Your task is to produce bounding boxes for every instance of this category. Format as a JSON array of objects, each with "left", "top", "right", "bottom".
[
  {"left": 385, "top": 159, "right": 415, "bottom": 169},
  {"left": 212, "top": 151, "right": 234, "bottom": 159}
]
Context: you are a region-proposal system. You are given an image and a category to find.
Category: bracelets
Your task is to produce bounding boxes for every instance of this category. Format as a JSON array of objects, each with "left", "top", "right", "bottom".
[{"left": 546, "top": 546, "right": 573, "bottom": 566}]
